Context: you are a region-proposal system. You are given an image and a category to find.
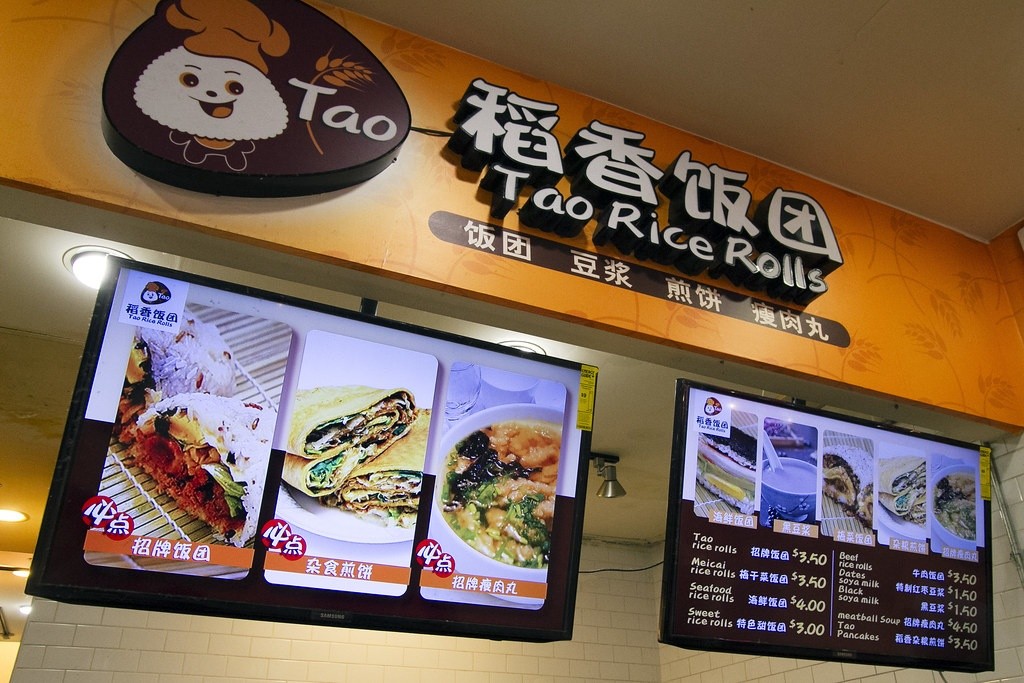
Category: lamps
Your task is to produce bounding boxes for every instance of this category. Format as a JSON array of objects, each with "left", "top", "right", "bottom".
[{"left": 595, "top": 466, "right": 626, "bottom": 499}]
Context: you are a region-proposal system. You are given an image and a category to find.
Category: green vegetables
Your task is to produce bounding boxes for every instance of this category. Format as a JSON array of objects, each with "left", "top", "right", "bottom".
[
  {"left": 939, "top": 500, "right": 977, "bottom": 540},
  {"left": 441, "top": 441, "right": 549, "bottom": 570}
]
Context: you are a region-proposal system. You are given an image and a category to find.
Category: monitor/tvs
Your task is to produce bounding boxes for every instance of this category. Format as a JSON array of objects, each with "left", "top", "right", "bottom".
[
  {"left": 658, "top": 377, "right": 994, "bottom": 674},
  {"left": 25, "top": 255, "right": 600, "bottom": 646}
]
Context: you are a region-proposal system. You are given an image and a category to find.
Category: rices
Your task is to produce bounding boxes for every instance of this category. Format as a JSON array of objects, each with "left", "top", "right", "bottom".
[{"left": 132, "top": 303, "right": 277, "bottom": 546}]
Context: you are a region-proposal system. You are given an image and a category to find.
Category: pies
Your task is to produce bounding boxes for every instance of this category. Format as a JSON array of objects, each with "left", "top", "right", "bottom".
[
  {"left": 281, "top": 383, "right": 418, "bottom": 496},
  {"left": 875, "top": 453, "right": 928, "bottom": 526},
  {"left": 320, "top": 408, "right": 432, "bottom": 528}
]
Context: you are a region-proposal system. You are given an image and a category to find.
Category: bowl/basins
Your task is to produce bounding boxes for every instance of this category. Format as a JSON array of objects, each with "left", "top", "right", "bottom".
[
  {"left": 761, "top": 457, "right": 817, "bottom": 522},
  {"left": 431, "top": 402, "right": 565, "bottom": 606},
  {"left": 931, "top": 464, "right": 976, "bottom": 551}
]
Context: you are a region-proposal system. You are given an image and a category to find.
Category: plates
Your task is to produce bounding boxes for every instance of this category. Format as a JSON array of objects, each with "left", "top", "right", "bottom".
[
  {"left": 276, "top": 482, "right": 414, "bottom": 545},
  {"left": 877, "top": 501, "right": 926, "bottom": 541}
]
